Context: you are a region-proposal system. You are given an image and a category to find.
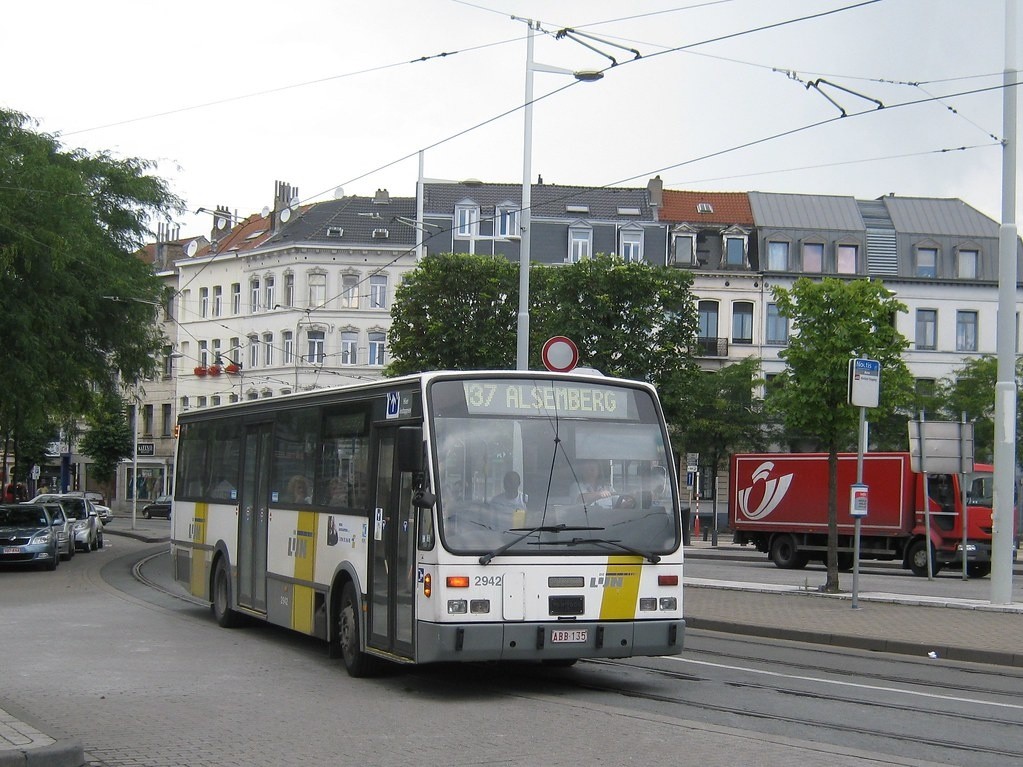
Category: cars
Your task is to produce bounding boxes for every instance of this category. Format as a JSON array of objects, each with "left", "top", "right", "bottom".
[
  {"left": 0, "top": 483, "right": 115, "bottom": 552},
  {"left": 0, "top": 505, "right": 60, "bottom": 571},
  {"left": 142, "top": 495, "right": 172, "bottom": 520},
  {"left": 42, "top": 503, "right": 76, "bottom": 561}
]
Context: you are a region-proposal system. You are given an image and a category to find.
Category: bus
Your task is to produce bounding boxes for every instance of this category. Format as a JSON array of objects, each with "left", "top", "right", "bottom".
[{"left": 171, "top": 369, "right": 687, "bottom": 679}]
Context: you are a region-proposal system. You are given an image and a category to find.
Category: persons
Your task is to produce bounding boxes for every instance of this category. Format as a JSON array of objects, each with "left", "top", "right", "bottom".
[
  {"left": 329, "top": 477, "right": 342, "bottom": 497},
  {"left": 569, "top": 459, "right": 634, "bottom": 509},
  {"left": 35, "top": 483, "right": 48, "bottom": 497},
  {"left": 286, "top": 475, "right": 312, "bottom": 504},
  {"left": 489, "top": 471, "right": 528, "bottom": 512}
]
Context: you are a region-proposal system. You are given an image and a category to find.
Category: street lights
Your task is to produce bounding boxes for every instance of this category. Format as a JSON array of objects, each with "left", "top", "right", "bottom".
[
  {"left": 131, "top": 353, "right": 183, "bottom": 528},
  {"left": 515, "top": 22, "right": 605, "bottom": 370}
]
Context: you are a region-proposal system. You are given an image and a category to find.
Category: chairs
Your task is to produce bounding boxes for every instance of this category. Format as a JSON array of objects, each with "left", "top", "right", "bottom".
[{"left": 214, "top": 459, "right": 578, "bottom": 518}]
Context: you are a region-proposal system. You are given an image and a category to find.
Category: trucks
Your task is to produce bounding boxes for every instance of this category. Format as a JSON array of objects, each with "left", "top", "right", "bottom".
[{"left": 729, "top": 452, "right": 1017, "bottom": 579}]
